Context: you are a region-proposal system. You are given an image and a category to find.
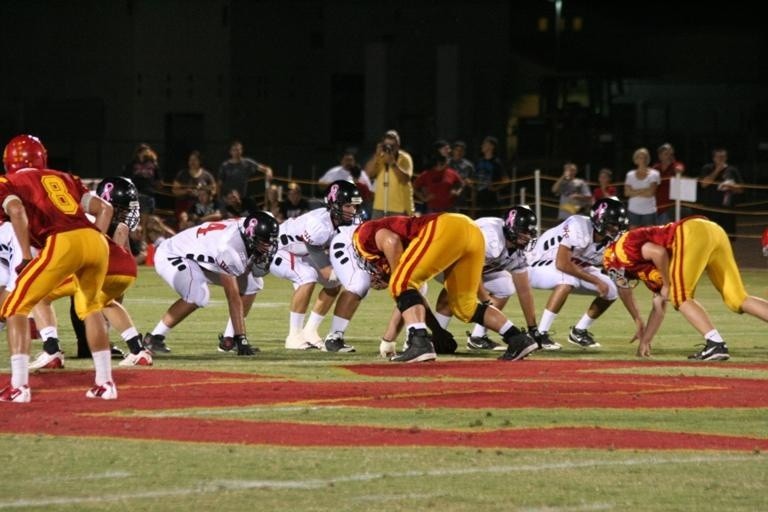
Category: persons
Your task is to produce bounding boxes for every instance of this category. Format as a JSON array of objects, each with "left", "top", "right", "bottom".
[{"left": 1, "top": 131, "right": 767, "bottom": 403}]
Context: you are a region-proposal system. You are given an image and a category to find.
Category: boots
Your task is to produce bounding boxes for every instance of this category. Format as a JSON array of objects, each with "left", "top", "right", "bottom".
[{"left": 390, "top": 327, "right": 437, "bottom": 362}]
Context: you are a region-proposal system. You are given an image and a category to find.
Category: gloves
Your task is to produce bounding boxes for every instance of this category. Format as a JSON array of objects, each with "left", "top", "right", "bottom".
[{"left": 232, "top": 334, "right": 253, "bottom": 356}]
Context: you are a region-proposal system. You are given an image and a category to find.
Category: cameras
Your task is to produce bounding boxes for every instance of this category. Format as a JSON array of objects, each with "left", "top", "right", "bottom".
[{"left": 382, "top": 143, "right": 392, "bottom": 153}]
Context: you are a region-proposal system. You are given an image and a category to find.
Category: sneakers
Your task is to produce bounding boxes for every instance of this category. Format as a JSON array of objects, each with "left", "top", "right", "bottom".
[
  {"left": 285, "top": 331, "right": 355, "bottom": 352},
  {"left": 218, "top": 333, "right": 259, "bottom": 352},
  {"left": 0, "top": 384, "right": 30, "bottom": 403},
  {"left": 85, "top": 382, "right": 117, "bottom": 400},
  {"left": 29, "top": 352, "right": 63, "bottom": 369},
  {"left": 111, "top": 332, "right": 170, "bottom": 366},
  {"left": 465, "top": 327, "right": 600, "bottom": 361},
  {"left": 689, "top": 341, "right": 730, "bottom": 362}
]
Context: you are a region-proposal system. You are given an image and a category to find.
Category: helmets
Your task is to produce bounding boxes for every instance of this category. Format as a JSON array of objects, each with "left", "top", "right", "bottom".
[
  {"left": 326, "top": 180, "right": 363, "bottom": 226},
  {"left": 245, "top": 209, "right": 278, "bottom": 271},
  {"left": 96, "top": 176, "right": 140, "bottom": 232},
  {"left": 590, "top": 196, "right": 629, "bottom": 243},
  {"left": 503, "top": 206, "right": 537, "bottom": 252}
]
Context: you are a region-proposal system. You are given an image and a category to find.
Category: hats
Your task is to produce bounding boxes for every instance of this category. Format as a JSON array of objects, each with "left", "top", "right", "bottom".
[{"left": 4, "top": 134, "right": 47, "bottom": 170}]
